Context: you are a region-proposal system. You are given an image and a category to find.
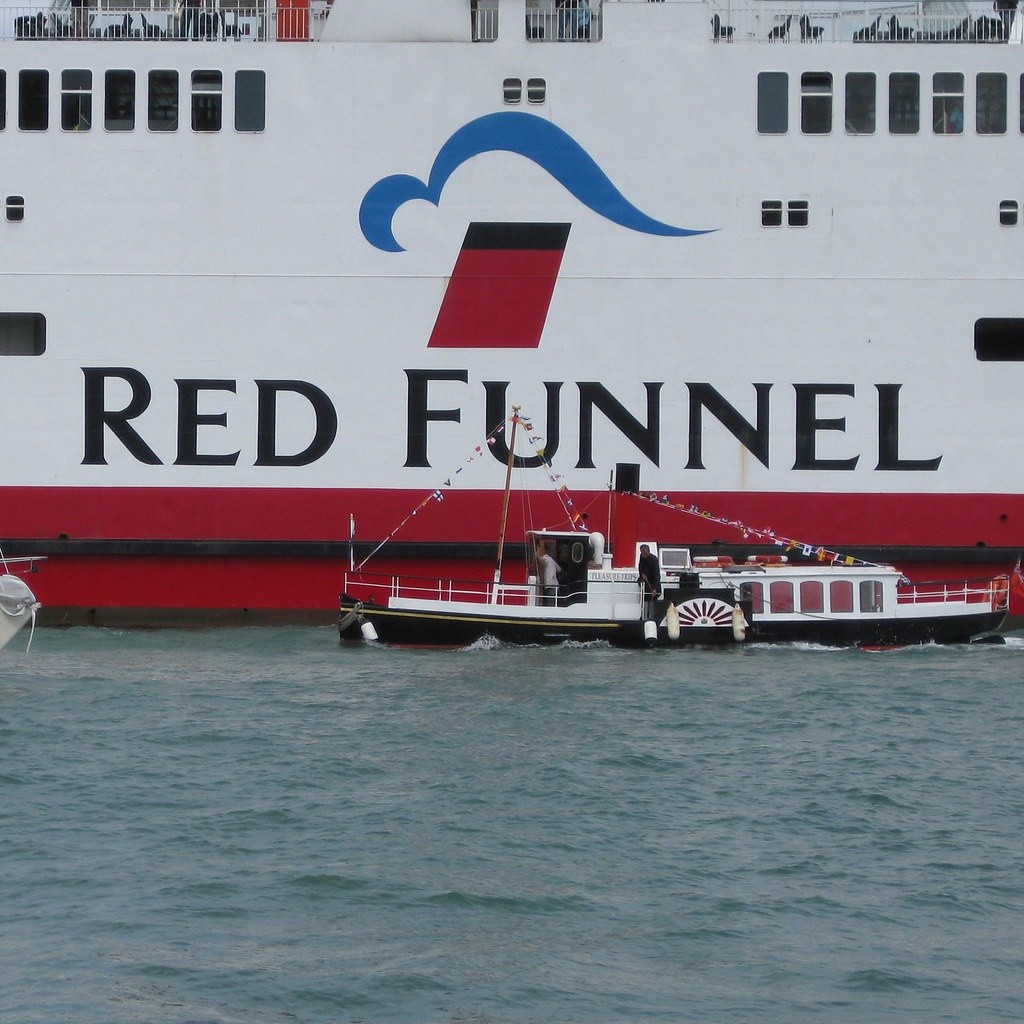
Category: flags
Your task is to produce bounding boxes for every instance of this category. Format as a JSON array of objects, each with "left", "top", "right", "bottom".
[
  {"left": 381, "top": 409, "right": 592, "bottom": 547},
  {"left": 350, "top": 519, "right": 357, "bottom": 555},
  {"left": 616, "top": 484, "right": 877, "bottom": 568}
]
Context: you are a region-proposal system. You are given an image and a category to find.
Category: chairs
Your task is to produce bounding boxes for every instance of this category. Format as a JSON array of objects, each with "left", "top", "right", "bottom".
[
  {"left": 767, "top": 15, "right": 825, "bottom": 44},
  {"left": 710, "top": 14, "right": 736, "bottom": 43},
  {"left": 526, "top": 14, "right": 546, "bottom": 42},
  {"left": 853, "top": 15, "right": 1006, "bottom": 43},
  {"left": 11, "top": 11, "right": 166, "bottom": 41}
]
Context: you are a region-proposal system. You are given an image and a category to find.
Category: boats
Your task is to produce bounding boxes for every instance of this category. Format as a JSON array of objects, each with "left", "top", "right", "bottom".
[{"left": 336, "top": 401, "right": 1012, "bottom": 646}]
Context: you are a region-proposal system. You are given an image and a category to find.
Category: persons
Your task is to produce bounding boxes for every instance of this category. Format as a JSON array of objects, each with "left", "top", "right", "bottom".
[
  {"left": 66, "top": 0, "right": 90, "bottom": 39},
  {"left": 637, "top": 544, "right": 662, "bottom": 621},
  {"left": 944, "top": 99, "right": 965, "bottom": 133},
  {"left": 555, "top": 0, "right": 593, "bottom": 43},
  {"left": 536, "top": 548, "right": 563, "bottom": 607},
  {"left": 177, "top": 0, "right": 203, "bottom": 41},
  {"left": 993, "top": 1, "right": 1019, "bottom": 42}
]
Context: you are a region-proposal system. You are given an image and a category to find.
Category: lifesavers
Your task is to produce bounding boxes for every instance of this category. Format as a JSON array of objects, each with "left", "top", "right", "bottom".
[
  {"left": 748, "top": 555, "right": 789, "bottom": 562},
  {"left": 693, "top": 555, "right": 733, "bottom": 567}
]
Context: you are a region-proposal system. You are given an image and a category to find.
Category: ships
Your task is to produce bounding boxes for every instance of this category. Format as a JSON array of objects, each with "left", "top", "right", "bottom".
[{"left": 0, "top": 0, "right": 1024, "bottom": 627}]
